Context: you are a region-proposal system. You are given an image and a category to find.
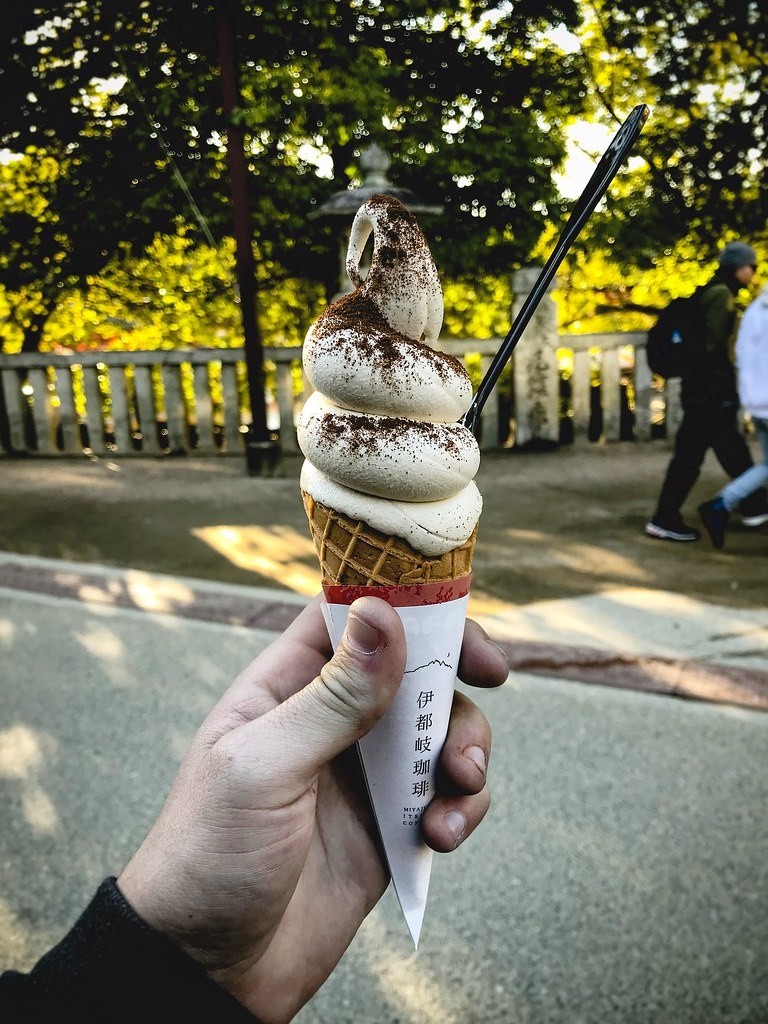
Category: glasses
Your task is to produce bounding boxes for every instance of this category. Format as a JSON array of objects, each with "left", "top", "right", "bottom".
[{"left": 750, "top": 265, "right": 758, "bottom": 273}]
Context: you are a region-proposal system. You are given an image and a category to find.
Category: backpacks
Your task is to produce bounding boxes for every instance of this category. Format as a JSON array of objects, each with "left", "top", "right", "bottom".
[{"left": 646, "top": 280, "right": 737, "bottom": 379}]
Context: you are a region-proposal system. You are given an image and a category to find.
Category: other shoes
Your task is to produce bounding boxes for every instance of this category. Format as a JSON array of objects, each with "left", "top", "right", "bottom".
[
  {"left": 698, "top": 497, "right": 730, "bottom": 549},
  {"left": 644, "top": 513, "right": 699, "bottom": 541},
  {"left": 741, "top": 501, "right": 768, "bottom": 526}
]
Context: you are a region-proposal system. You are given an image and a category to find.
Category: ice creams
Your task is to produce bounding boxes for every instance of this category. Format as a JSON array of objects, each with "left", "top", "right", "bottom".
[{"left": 295, "top": 194, "right": 483, "bottom": 953}]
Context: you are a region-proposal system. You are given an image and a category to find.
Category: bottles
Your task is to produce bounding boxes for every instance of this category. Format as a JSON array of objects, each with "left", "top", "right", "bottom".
[{"left": 672, "top": 331, "right": 681, "bottom": 343}]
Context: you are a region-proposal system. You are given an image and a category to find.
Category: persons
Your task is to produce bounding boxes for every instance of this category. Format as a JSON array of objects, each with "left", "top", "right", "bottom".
[
  {"left": 699, "top": 288, "right": 768, "bottom": 550},
  {"left": 0, "top": 583, "right": 510, "bottom": 1024},
  {"left": 644, "top": 244, "right": 768, "bottom": 540}
]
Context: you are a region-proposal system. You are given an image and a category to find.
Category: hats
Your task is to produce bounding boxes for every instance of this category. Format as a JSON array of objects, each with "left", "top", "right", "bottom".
[{"left": 719, "top": 243, "right": 756, "bottom": 266}]
